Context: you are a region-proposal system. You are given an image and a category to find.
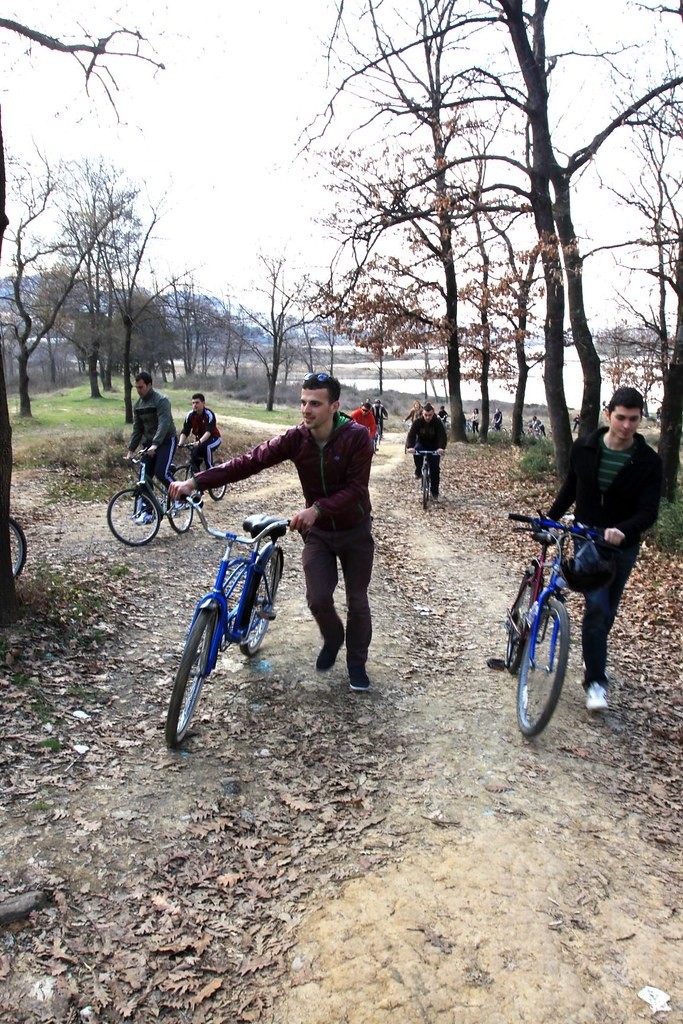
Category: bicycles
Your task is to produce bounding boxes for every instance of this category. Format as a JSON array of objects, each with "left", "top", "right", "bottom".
[
  {"left": 168, "top": 441, "right": 229, "bottom": 502},
  {"left": 107, "top": 442, "right": 194, "bottom": 547},
  {"left": 9, "top": 519, "right": 29, "bottom": 580},
  {"left": 405, "top": 449, "right": 442, "bottom": 510},
  {"left": 484, "top": 510, "right": 626, "bottom": 740},
  {"left": 163, "top": 489, "right": 292, "bottom": 744}
]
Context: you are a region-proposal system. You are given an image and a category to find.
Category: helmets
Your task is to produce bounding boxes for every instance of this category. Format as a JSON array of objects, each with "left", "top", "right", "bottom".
[
  {"left": 375, "top": 399, "right": 381, "bottom": 403},
  {"left": 561, "top": 538, "right": 613, "bottom": 592}
]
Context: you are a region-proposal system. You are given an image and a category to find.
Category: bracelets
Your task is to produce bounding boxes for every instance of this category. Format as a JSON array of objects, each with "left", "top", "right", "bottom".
[{"left": 198, "top": 440, "right": 201, "bottom": 445}]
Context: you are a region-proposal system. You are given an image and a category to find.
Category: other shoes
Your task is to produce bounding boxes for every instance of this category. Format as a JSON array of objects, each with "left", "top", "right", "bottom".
[
  {"left": 175, "top": 500, "right": 184, "bottom": 510},
  {"left": 430, "top": 487, "right": 438, "bottom": 502},
  {"left": 415, "top": 474, "right": 419, "bottom": 479},
  {"left": 585, "top": 681, "right": 608, "bottom": 710},
  {"left": 347, "top": 662, "right": 370, "bottom": 691},
  {"left": 135, "top": 511, "right": 153, "bottom": 526},
  {"left": 316, "top": 634, "right": 345, "bottom": 670}
]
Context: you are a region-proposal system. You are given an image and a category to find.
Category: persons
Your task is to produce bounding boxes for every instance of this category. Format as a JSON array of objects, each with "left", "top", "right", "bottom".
[
  {"left": 543, "top": 386, "right": 665, "bottom": 709},
  {"left": 532, "top": 416, "right": 546, "bottom": 436},
  {"left": 350, "top": 403, "right": 376, "bottom": 443},
  {"left": 402, "top": 400, "right": 423, "bottom": 425},
  {"left": 372, "top": 400, "right": 388, "bottom": 438},
  {"left": 655, "top": 409, "right": 661, "bottom": 428},
  {"left": 167, "top": 373, "right": 374, "bottom": 690},
  {"left": 177, "top": 393, "right": 222, "bottom": 495},
  {"left": 437, "top": 406, "right": 447, "bottom": 423},
  {"left": 127, "top": 372, "right": 185, "bottom": 524},
  {"left": 573, "top": 412, "right": 579, "bottom": 431},
  {"left": 491, "top": 408, "right": 502, "bottom": 431},
  {"left": 468, "top": 408, "right": 479, "bottom": 434},
  {"left": 404, "top": 403, "right": 447, "bottom": 502},
  {"left": 365, "top": 398, "right": 374, "bottom": 416},
  {"left": 601, "top": 401, "right": 605, "bottom": 413}
]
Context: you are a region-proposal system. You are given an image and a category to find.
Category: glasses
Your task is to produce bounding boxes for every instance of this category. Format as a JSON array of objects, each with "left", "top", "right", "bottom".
[
  {"left": 304, "top": 372, "right": 329, "bottom": 382},
  {"left": 362, "top": 409, "right": 368, "bottom": 413}
]
